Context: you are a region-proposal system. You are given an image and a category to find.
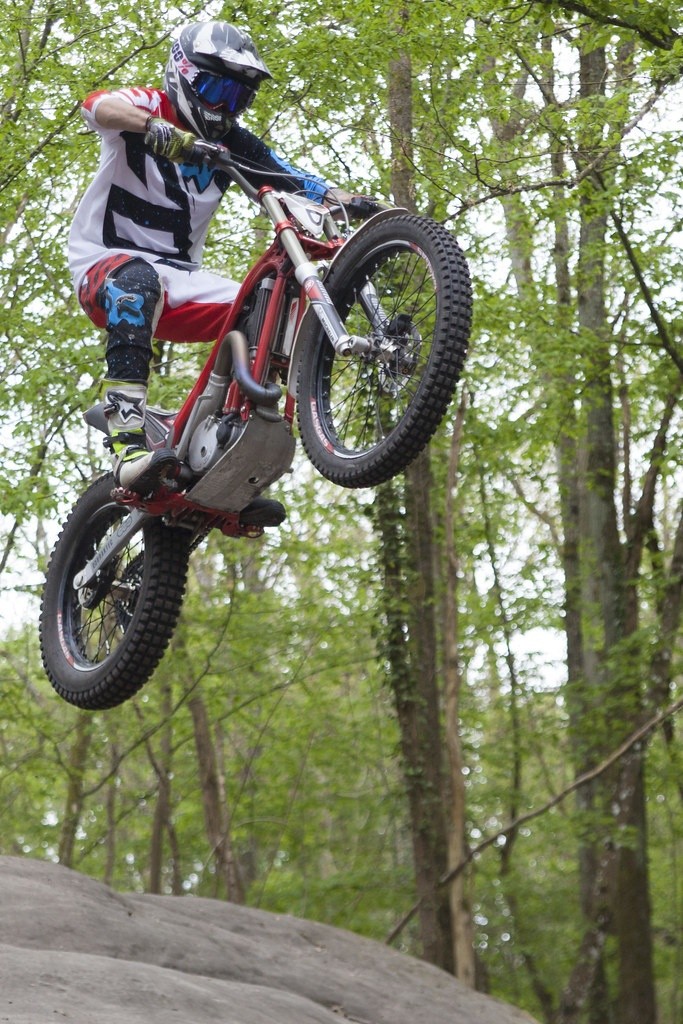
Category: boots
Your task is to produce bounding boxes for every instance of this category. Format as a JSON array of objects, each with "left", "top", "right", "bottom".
[
  {"left": 98, "top": 377, "right": 181, "bottom": 501},
  {"left": 239, "top": 498, "right": 286, "bottom": 527}
]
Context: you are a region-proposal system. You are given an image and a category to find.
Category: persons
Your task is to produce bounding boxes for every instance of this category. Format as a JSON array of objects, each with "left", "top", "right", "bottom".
[{"left": 67, "top": 21, "right": 398, "bottom": 526}]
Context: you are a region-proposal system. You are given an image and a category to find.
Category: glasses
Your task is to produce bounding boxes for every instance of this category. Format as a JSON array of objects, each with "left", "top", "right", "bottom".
[{"left": 190, "top": 69, "right": 256, "bottom": 117}]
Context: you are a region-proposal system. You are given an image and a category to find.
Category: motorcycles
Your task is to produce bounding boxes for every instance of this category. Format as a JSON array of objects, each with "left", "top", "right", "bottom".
[{"left": 37, "top": 124, "right": 474, "bottom": 713}]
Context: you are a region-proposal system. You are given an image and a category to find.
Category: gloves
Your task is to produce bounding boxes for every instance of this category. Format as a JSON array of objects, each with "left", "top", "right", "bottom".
[
  {"left": 360, "top": 199, "right": 397, "bottom": 223},
  {"left": 143, "top": 117, "right": 197, "bottom": 165}
]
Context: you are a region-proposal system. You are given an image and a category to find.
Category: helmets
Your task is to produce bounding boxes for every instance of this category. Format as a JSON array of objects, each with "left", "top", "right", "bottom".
[{"left": 163, "top": 21, "right": 273, "bottom": 144}]
